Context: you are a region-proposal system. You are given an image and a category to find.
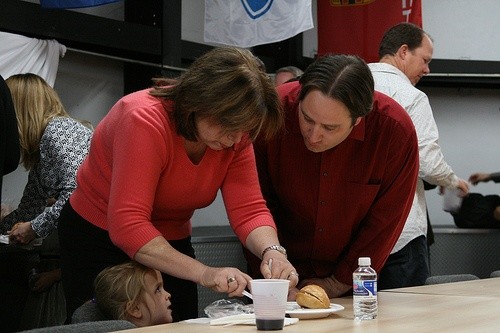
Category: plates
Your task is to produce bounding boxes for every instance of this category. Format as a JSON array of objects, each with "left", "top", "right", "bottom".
[{"left": 286, "top": 299, "right": 343, "bottom": 319}]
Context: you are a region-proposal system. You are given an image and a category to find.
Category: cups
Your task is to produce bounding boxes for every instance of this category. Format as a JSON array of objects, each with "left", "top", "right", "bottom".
[{"left": 248, "top": 279, "right": 290, "bottom": 330}]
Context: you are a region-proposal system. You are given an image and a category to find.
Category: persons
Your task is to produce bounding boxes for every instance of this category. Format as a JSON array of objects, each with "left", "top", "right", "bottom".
[
  {"left": 0, "top": 73, "right": 95, "bottom": 295},
  {"left": 58, "top": 46, "right": 299, "bottom": 325},
  {"left": 242, "top": 52, "right": 420, "bottom": 305},
  {"left": 83, "top": 261, "right": 173, "bottom": 327},
  {"left": 367, "top": 22, "right": 468, "bottom": 290}
]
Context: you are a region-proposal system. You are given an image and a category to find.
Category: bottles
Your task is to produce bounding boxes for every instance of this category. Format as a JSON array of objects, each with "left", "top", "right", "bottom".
[{"left": 353, "top": 257, "right": 378, "bottom": 321}]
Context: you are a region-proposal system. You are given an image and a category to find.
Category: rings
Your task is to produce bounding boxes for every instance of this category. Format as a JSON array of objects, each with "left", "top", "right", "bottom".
[
  {"left": 291, "top": 272, "right": 299, "bottom": 277},
  {"left": 227, "top": 277, "right": 236, "bottom": 283}
]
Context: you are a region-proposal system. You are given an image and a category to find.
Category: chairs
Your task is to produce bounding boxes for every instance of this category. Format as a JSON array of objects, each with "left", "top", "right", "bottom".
[
  {"left": 425, "top": 274, "right": 479, "bottom": 285},
  {"left": 21, "top": 320, "right": 137, "bottom": 333},
  {"left": 490, "top": 271, "right": 500, "bottom": 278}
]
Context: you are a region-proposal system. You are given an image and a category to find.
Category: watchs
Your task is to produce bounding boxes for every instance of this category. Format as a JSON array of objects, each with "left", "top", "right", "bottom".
[{"left": 262, "top": 245, "right": 287, "bottom": 260}]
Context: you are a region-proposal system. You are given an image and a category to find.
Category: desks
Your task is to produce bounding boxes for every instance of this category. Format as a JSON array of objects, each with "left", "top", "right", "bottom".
[{"left": 111, "top": 277, "right": 500, "bottom": 332}]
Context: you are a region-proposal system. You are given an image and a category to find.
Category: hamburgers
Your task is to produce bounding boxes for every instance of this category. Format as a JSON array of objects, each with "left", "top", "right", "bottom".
[{"left": 296, "top": 284, "right": 330, "bottom": 309}]
